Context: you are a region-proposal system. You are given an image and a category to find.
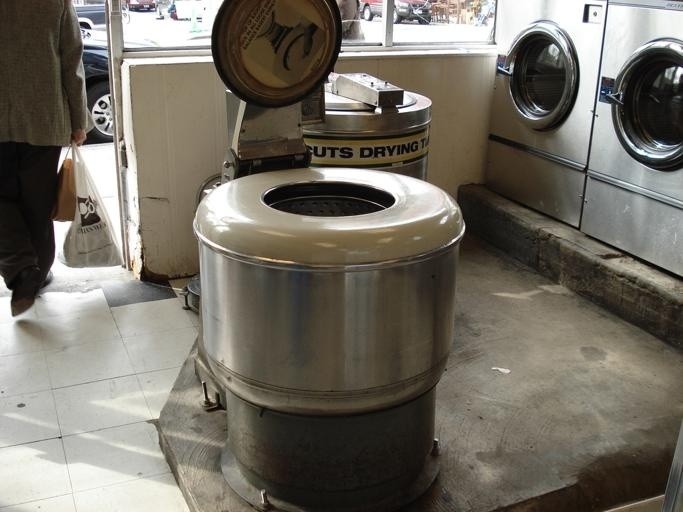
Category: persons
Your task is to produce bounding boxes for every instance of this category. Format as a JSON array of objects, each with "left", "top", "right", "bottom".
[
  {"left": 335, "top": 0, "right": 366, "bottom": 40},
  {"left": 1, "top": 1, "right": 88, "bottom": 317}
]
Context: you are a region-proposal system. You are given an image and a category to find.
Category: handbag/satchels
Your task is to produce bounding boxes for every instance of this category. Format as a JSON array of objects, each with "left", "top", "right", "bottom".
[{"left": 48, "top": 159, "right": 76, "bottom": 222}]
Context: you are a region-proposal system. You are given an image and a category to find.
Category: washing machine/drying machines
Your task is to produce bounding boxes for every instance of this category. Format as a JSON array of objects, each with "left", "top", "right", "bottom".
[
  {"left": 579, "top": 1, "right": 682, "bottom": 282},
  {"left": 483, "top": 0, "right": 607, "bottom": 229}
]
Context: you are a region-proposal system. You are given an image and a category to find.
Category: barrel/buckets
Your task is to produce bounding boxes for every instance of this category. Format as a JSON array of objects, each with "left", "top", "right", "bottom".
[{"left": 299, "top": 75, "right": 434, "bottom": 182}]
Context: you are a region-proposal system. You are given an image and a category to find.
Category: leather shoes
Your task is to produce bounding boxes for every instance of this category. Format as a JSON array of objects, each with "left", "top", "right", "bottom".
[{"left": 11, "top": 265, "right": 53, "bottom": 316}]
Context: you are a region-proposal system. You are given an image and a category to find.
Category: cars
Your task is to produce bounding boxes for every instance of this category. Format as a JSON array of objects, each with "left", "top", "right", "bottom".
[{"left": 72, "top": 1, "right": 207, "bottom": 144}]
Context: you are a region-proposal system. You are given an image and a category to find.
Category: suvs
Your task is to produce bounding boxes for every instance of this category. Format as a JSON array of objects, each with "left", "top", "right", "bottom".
[{"left": 358, "top": 0, "right": 432, "bottom": 25}]
